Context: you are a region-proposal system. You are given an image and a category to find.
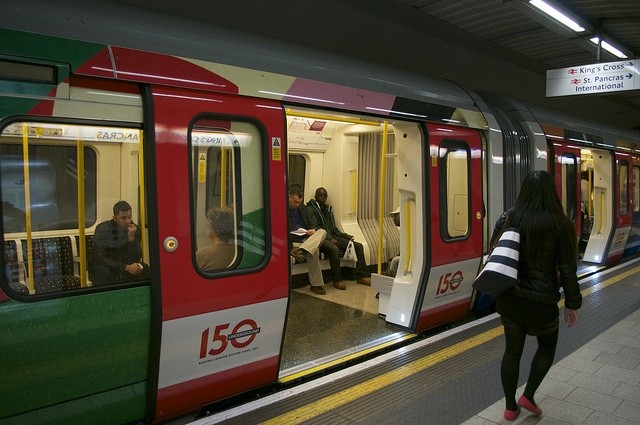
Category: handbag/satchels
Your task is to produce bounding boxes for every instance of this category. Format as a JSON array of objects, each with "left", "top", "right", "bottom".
[{"left": 471, "top": 208, "right": 522, "bottom": 298}]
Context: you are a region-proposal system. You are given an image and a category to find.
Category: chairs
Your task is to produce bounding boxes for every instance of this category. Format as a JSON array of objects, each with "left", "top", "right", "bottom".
[
  {"left": 4, "top": 228, "right": 30, "bottom": 294},
  {"left": 74, "top": 235, "right": 143, "bottom": 286},
  {"left": 20, "top": 232, "right": 84, "bottom": 294}
]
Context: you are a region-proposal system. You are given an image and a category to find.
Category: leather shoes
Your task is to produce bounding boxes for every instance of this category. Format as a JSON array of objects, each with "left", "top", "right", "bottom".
[
  {"left": 291, "top": 250, "right": 306, "bottom": 262},
  {"left": 311, "top": 285, "right": 326, "bottom": 295},
  {"left": 334, "top": 280, "right": 346, "bottom": 289},
  {"left": 357, "top": 277, "right": 372, "bottom": 285},
  {"left": 518, "top": 394, "right": 543, "bottom": 414},
  {"left": 505, "top": 406, "right": 521, "bottom": 421}
]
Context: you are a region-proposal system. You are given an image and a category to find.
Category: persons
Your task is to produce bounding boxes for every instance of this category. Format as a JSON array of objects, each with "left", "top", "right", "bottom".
[
  {"left": 490, "top": 170, "right": 582, "bottom": 421},
  {"left": 578, "top": 171, "right": 592, "bottom": 260},
  {"left": 87, "top": 201, "right": 152, "bottom": 287},
  {"left": 306, "top": 187, "right": 371, "bottom": 290},
  {"left": 288, "top": 184, "right": 327, "bottom": 295},
  {"left": 195, "top": 206, "right": 235, "bottom": 272}
]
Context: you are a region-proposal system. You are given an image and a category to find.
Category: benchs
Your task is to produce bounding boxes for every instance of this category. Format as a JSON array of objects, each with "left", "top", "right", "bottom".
[{"left": 257, "top": 211, "right": 339, "bottom": 272}]
